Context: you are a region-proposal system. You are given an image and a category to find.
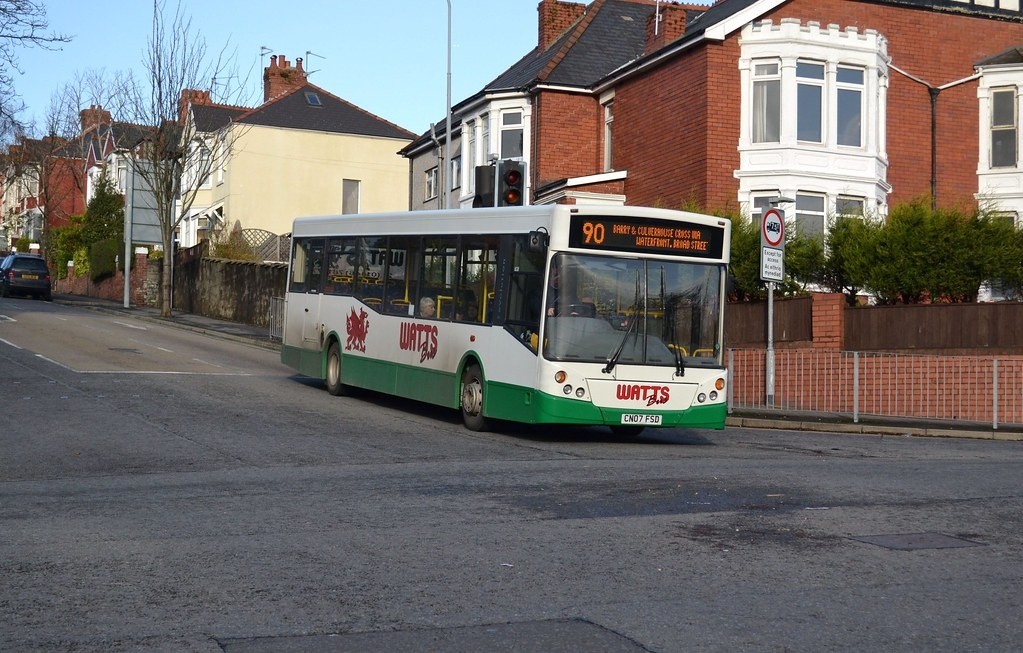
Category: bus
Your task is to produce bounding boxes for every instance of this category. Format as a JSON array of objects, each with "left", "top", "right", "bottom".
[{"left": 281, "top": 203, "right": 739, "bottom": 437}]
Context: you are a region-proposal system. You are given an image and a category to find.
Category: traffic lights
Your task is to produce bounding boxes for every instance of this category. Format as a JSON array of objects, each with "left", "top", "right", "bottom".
[{"left": 492, "top": 158, "right": 530, "bottom": 206}]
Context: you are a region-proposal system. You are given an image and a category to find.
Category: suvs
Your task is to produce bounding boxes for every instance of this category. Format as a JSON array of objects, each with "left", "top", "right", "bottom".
[{"left": 0, "top": 252, "right": 52, "bottom": 301}]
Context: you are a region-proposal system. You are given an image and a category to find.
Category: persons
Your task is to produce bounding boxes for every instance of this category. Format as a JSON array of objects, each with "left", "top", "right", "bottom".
[
  {"left": 530, "top": 263, "right": 586, "bottom": 326},
  {"left": 419, "top": 296, "right": 436, "bottom": 317}
]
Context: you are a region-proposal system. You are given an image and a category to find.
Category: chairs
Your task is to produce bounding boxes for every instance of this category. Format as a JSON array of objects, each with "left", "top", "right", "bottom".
[{"left": 330, "top": 274, "right": 494, "bottom": 323}]
